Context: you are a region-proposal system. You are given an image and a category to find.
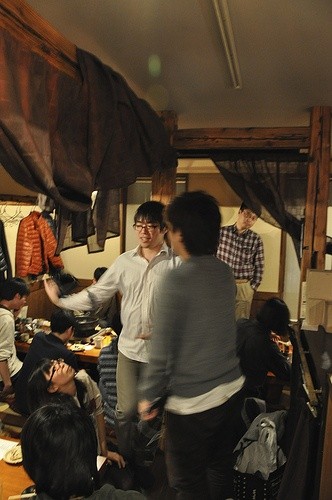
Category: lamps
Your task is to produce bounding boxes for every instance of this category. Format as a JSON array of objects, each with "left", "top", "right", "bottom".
[{"left": 213, "top": 0, "right": 242, "bottom": 89}]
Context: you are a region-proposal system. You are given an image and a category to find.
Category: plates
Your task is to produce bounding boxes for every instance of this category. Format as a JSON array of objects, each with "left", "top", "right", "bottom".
[{"left": 4, "top": 446, "right": 23, "bottom": 463}]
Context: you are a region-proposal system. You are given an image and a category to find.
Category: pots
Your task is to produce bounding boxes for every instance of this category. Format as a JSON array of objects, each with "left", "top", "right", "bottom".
[{"left": 74, "top": 316, "right": 100, "bottom": 331}]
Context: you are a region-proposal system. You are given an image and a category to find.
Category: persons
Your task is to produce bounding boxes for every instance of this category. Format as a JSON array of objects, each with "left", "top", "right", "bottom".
[
  {"left": 216, "top": 200, "right": 265, "bottom": 322},
  {"left": 0, "top": 267, "right": 293, "bottom": 500},
  {"left": 136, "top": 189, "right": 246, "bottom": 500},
  {"left": 41, "top": 200, "right": 183, "bottom": 460}
]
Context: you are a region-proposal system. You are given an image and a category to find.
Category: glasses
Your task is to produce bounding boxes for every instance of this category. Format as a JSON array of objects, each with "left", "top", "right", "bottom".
[
  {"left": 132, "top": 223, "right": 160, "bottom": 231},
  {"left": 46, "top": 357, "right": 65, "bottom": 387}
]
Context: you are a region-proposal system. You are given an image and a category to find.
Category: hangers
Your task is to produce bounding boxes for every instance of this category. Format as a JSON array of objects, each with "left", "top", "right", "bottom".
[{"left": 0, "top": 202, "right": 38, "bottom": 223}]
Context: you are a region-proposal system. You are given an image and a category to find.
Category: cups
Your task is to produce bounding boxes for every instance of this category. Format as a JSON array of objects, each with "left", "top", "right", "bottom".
[{"left": 95, "top": 336, "right": 102, "bottom": 349}]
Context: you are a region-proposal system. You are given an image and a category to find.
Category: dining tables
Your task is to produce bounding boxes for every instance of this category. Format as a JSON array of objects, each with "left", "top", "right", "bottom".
[
  {"left": 0, "top": 435, "right": 109, "bottom": 500},
  {"left": 13, "top": 315, "right": 117, "bottom": 365}
]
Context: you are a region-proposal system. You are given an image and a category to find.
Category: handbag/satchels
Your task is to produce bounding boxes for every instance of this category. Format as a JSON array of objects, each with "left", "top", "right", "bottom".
[{"left": 229, "top": 396, "right": 289, "bottom": 500}]
